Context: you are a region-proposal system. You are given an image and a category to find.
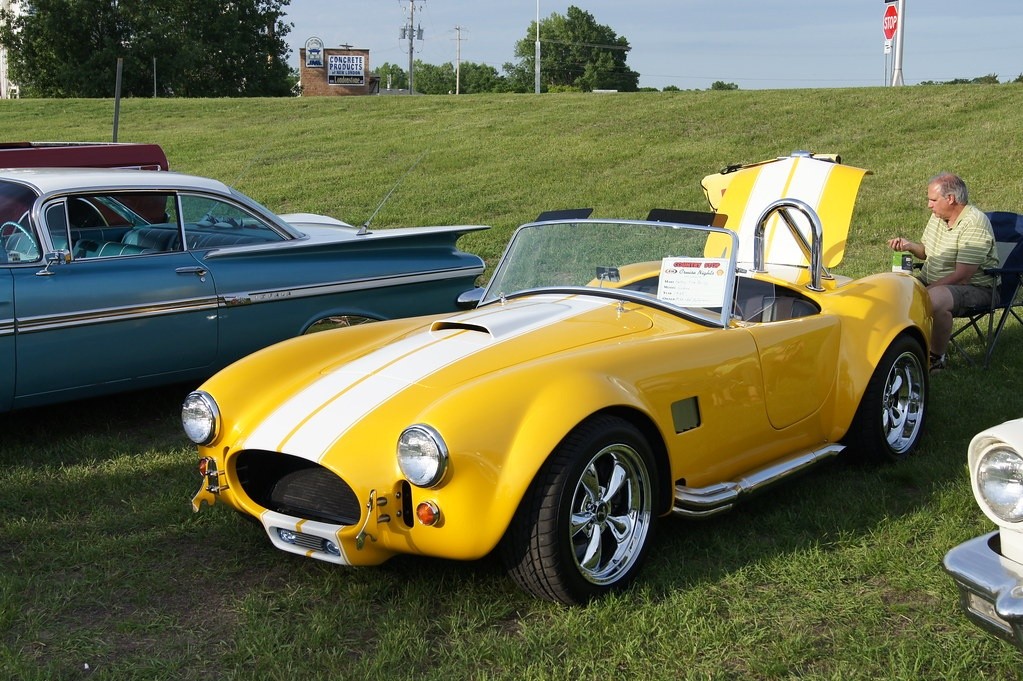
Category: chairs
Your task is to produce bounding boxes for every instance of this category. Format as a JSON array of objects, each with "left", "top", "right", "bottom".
[
  {"left": 915, "top": 211, "right": 1023, "bottom": 369},
  {"left": 4, "top": 224, "right": 275, "bottom": 264}
]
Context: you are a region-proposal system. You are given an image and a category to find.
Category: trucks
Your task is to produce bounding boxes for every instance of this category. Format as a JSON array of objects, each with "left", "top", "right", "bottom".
[{"left": 0, "top": 137, "right": 171, "bottom": 233}]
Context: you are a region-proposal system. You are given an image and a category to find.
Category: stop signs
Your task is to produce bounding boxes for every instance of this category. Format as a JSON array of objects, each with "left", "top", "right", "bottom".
[{"left": 882, "top": 4, "right": 899, "bottom": 39}]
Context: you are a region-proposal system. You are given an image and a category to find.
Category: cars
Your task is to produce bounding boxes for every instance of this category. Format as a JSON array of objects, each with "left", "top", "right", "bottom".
[
  {"left": 940, "top": 416, "right": 1023, "bottom": 654},
  {"left": 0, "top": 166, "right": 490, "bottom": 413},
  {"left": 180, "top": 151, "right": 935, "bottom": 617}
]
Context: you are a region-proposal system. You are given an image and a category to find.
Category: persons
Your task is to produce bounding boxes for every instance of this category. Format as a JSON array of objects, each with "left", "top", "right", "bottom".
[{"left": 885, "top": 172, "right": 1003, "bottom": 376}]
